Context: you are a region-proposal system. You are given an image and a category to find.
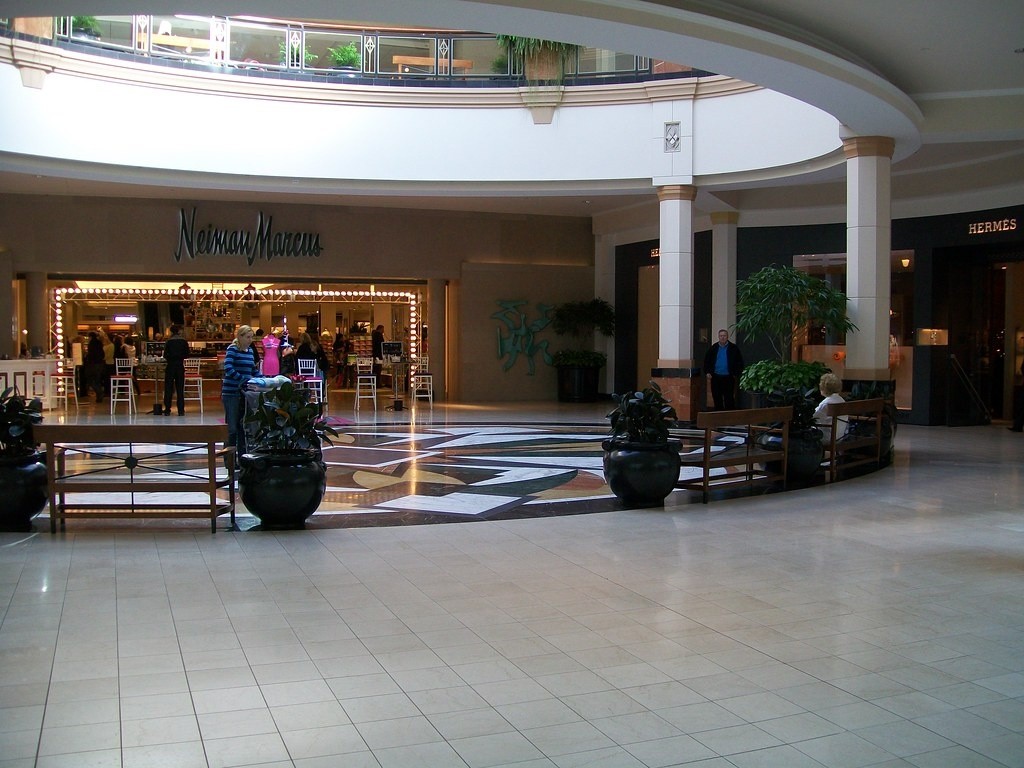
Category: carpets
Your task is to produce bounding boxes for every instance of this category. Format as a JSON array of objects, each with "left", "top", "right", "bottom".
[{"left": 313, "top": 416, "right": 355, "bottom": 425}]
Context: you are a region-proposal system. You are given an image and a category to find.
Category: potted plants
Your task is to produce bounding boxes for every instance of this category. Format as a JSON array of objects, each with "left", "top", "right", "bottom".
[
  {"left": 0, "top": 386, "right": 54, "bottom": 531},
  {"left": 60, "top": 16, "right": 104, "bottom": 49},
  {"left": 495, "top": 34, "right": 586, "bottom": 111},
  {"left": 277, "top": 42, "right": 319, "bottom": 73},
  {"left": 237, "top": 381, "right": 339, "bottom": 525},
  {"left": 602, "top": 380, "right": 684, "bottom": 508},
  {"left": 326, "top": 41, "right": 368, "bottom": 77},
  {"left": 727, "top": 261, "right": 898, "bottom": 477},
  {"left": 550, "top": 297, "right": 617, "bottom": 401}
]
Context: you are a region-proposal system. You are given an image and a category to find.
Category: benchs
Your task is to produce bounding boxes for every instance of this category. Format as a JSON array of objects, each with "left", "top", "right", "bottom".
[
  {"left": 821, "top": 397, "right": 886, "bottom": 481},
  {"left": 674, "top": 406, "right": 794, "bottom": 505},
  {"left": 31, "top": 423, "right": 236, "bottom": 534},
  {"left": 392, "top": 55, "right": 473, "bottom": 80},
  {"left": 137, "top": 32, "right": 225, "bottom": 60}
]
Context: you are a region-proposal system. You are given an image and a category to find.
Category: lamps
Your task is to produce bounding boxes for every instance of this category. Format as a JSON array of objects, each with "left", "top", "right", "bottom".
[
  {"left": 179, "top": 282, "right": 190, "bottom": 289},
  {"left": 244, "top": 283, "right": 256, "bottom": 291}
]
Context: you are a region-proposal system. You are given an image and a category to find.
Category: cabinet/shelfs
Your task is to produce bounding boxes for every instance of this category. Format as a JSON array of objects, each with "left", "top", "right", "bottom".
[{"left": 135, "top": 301, "right": 411, "bottom": 381}]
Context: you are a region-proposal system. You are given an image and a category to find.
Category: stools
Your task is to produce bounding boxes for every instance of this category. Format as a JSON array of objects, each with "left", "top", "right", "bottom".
[
  {"left": 109, "top": 358, "right": 136, "bottom": 415},
  {"left": 413, "top": 358, "right": 433, "bottom": 408},
  {"left": 354, "top": 358, "right": 377, "bottom": 412},
  {"left": 49, "top": 358, "right": 78, "bottom": 413},
  {"left": 183, "top": 358, "right": 205, "bottom": 414},
  {"left": 298, "top": 359, "right": 322, "bottom": 404}
]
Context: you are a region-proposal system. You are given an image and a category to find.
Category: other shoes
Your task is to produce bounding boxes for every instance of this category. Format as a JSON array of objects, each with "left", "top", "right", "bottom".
[
  {"left": 164, "top": 410, "right": 171, "bottom": 416},
  {"left": 178, "top": 410, "right": 184, "bottom": 416}
]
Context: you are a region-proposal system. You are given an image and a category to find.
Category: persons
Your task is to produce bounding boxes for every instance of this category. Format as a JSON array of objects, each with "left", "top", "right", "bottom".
[
  {"left": 65, "top": 332, "right": 143, "bottom": 402},
  {"left": 222, "top": 324, "right": 386, "bottom": 471},
  {"left": 704, "top": 329, "right": 743, "bottom": 411},
  {"left": 811, "top": 374, "right": 849, "bottom": 446},
  {"left": 163, "top": 324, "right": 192, "bottom": 416}
]
